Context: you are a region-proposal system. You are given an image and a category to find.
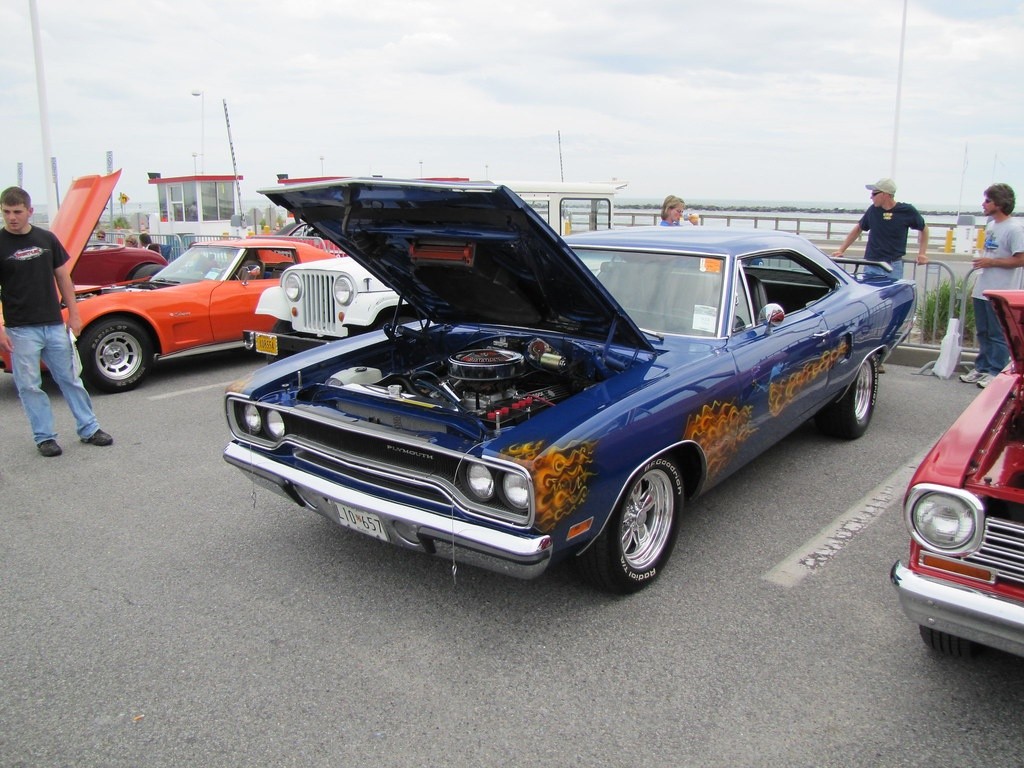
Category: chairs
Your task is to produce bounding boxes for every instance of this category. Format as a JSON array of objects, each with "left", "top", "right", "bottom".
[{"left": 596, "top": 261, "right": 768, "bottom": 331}]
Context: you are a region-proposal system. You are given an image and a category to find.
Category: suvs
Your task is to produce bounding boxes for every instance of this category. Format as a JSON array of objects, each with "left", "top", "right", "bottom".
[{"left": 244, "top": 255, "right": 424, "bottom": 365}]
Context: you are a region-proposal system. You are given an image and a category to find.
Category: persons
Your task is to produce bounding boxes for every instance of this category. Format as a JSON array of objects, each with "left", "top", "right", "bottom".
[
  {"left": 125, "top": 235, "right": 139, "bottom": 249},
  {"left": 0, "top": 187, "right": 114, "bottom": 460},
  {"left": 96, "top": 230, "right": 106, "bottom": 241},
  {"left": 830, "top": 178, "right": 929, "bottom": 373},
  {"left": 659, "top": 195, "right": 685, "bottom": 226},
  {"left": 960, "top": 183, "right": 1024, "bottom": 387},
  {"left": 190, "top": 201, "right": 199, "bottom": 220},
  {"left": 140, "top": 233, "right": 161, "bottom": 251}
]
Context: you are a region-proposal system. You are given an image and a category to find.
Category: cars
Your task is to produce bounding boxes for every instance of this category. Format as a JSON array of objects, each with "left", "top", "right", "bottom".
[
  {"left": 889, "top": 288, "right": 1024, "bottom": 659},
  {"left": 0, "top": 168, "right": 340, "bottom": 394},
  {"left": 223, "top": 177, "right": 914, "bottom": 595},
  {"left": 250, "top": 221, "right": 347, "bottom": 257}
]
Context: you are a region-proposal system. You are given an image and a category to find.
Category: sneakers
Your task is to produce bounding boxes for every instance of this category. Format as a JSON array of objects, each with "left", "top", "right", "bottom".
[
  {"left": 977, "top": 372, "right": 996, "bottom": 388},
  {"left": 38, "top": 440, "right": 62, "bottom": 457},
  {"left": 81, "top": 428, "right": 112, "bottom": 445},
  {"left": 959, "top": 368, "right": 985, "bottom": 384}
]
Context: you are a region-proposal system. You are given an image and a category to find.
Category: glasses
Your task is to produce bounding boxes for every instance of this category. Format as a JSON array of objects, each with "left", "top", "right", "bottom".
[{"left": 871, "top": 190, "right": 883, "bottom": 195}]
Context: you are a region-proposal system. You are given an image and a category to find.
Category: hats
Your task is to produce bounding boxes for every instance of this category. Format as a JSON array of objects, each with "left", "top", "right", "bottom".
[{"left": 866, "top": 177, "right": 896, "bottom": 196}]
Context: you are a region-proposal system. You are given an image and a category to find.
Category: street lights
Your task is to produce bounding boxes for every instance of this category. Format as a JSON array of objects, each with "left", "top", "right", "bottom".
[
  {"left": 419, "top": 161, "right": 423, "bottom": 178},
  {"left": 485, "top": 165, "right": 488, "bottom": 176},
  {"left": 192, "top": 89, "right": 206, "bottom": 175},
  {"left": 320, "top": 156, "right": 325, "bottom": 177}
]
